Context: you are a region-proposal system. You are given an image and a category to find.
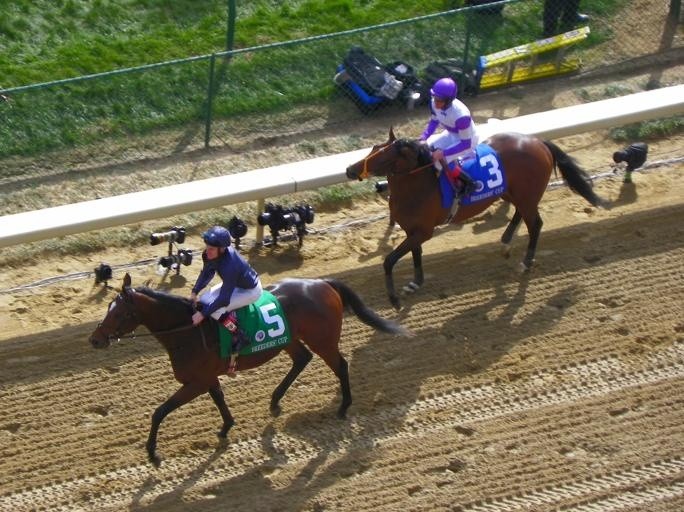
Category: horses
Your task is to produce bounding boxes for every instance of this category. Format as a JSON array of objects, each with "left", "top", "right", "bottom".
[
  {"left": 87, "top": 272, "right": 407, "bottom": 470},
  {"left": 344, "top": 124, "right": 602, "bottom": 313}
]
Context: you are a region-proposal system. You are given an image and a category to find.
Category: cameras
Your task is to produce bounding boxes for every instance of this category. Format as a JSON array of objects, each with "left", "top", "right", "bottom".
[
  {"left": 258, "top": 203, "right": 314, "bottom": 230},
  {"left": 614, "top": 143, "right": 648, "bottom": 169},
  {"left": 151, "top": 225, "right": 185, "bottom": 245},
  {"left": 95, "top": 265, "right": 112, "bottom": 281},
  {"left": 375, "top": 181, "right": 388, "bottom": 193},
  {"left": 228, "top": 218, "right": 247, "bottom": 238},
  {"left": 160, "top": 249, "right": 192, "bottom": 267}
]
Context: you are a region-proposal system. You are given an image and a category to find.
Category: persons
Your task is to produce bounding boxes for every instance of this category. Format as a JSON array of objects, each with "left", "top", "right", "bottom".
[
  {"left": 191, "top": 227, "right": 263, "bottom": 352},
  {"left": 416, "top": 78, "right": 480, "bottom": 199}
]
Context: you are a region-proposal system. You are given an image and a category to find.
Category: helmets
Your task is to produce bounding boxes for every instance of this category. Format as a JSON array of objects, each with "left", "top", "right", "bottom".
[
  {"left": 430, "top": 77, "right": 458, "bottom": 98},
  {"left": 204, "top": 226, "right": 231, "bottom": 246}
]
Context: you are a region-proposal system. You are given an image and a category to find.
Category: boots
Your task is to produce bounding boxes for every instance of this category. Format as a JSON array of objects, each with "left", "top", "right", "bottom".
[
  {"left": 458, "top": 170, "right": 477, "bottom": 198},
  {"left": 230, "top": 329, "right": 250, "bottom": 353}
]
